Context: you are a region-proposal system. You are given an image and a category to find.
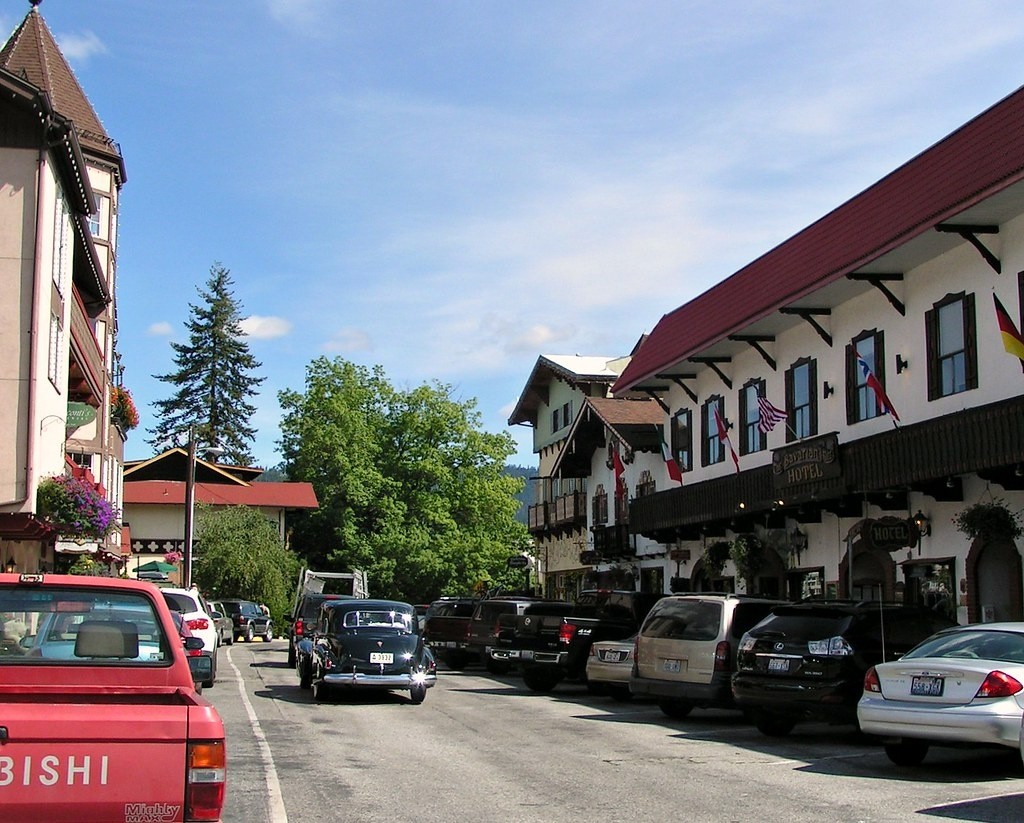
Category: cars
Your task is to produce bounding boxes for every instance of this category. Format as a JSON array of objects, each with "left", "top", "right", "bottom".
[
  {"left": 854, "top": 620, "right": 1024, "bottom": 774},
  {"left": 295, "top": 599, "right": 438, "bottom": 704}
]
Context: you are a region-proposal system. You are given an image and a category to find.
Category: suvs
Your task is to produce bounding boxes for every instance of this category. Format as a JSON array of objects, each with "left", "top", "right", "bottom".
[
  {"left": 410, "top": 590, "right": 671, "bottom": 703},
  {"left": 220, "top": 597, "right": 274, "bottom": 642},
  {"left": 729, "top": 593, "right": 964, "bottom": 746},
  {"left": 21, "top": 585, "right": 235, "bottom": 696},
  {"left": 282, "top": 592, "right": 358, "bottom": 670}
]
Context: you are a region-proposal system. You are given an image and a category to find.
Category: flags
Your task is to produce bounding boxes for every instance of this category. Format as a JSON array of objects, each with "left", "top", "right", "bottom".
[
  {"left": 850, "top": 341, "right": 900, "bottom": 434},
  {"left": 753, "top": 385, "right": 791, "bottom": 435},
  {"left": 993, "top": 292, "right": 1024, "bottom": 374},
  {"left": 712, "top": 402, "right": 740, "bottom": 480},
  {"left": 612, "top": 446, "right": 627, "bottom": 501},
  {"left": 656, "top": 426, "right": 684, "bottom": 488}
]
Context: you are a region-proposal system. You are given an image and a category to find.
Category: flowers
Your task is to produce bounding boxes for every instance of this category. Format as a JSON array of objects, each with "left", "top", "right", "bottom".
[
  {"left": 35, "top": 473, "right": 125, "bottom": 543},
  {"left": 109, "top": 382, "right": 141, "bottom": 430}
]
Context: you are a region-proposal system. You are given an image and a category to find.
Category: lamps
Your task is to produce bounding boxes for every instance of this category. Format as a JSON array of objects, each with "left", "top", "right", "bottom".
[
  {"left": 839, "top": 498, "right": 846, "bottom": 508},
  {"left": 5, "top": 557, "right": 16, "bottom": 573},
  {"left": 885, "top": 492, "right": 893, "bottom": 499},
  {"left": 823, "top": 382, "right": 834, "bottom": 399},
  {"left": 913, "top": 509, "right": 932, "bottom": 555},
  {"left": 1015, "top": 464, "right": 1023, "bottom": 476},
  {"left": 675, "top": 508, "right": 805, "bottom": 533},
  {"left": 896, "top": 355, "right": 907, "bottom": 374},
  {"left": 946, "top": 477, "right": 954, "bottom": 488},
  {"left": 723, "top": 418, "right": 733, "bottom": 432},
  {"left": 790, "top": 527, "right": 808, "bottom": 565},
  {"left": 631, "top": 563, "right": 639, "bottom": 580}
]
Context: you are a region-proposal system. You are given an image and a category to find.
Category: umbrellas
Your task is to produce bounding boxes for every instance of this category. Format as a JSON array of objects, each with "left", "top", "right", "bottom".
[{"left": 132, "top": 561, "right": 175, "bottom": 572}]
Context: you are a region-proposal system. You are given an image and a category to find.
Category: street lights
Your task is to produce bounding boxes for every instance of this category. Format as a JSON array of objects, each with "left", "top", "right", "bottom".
[{"left": 184, "top": 447, "right": 223, "bottom": 591}]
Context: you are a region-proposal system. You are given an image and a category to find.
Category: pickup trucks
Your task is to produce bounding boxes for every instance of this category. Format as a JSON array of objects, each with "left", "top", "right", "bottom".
[{"left": 0, "top": 569, "right": 226, "bottom": 823}]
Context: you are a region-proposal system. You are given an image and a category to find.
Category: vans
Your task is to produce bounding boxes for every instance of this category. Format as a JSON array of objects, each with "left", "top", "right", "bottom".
[{"left": 628, "top": 591, "right": 817, "bottom": 720}]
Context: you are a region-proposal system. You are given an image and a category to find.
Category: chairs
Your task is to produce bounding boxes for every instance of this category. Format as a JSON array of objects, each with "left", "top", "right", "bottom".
[{"left": 74, "top": 620, "right": 139, "bottom": 662}]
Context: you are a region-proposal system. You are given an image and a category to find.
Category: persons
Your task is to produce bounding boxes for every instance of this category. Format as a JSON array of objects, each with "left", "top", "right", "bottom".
[
  {"left": 190, "top": 582, "right": 200, "bottom": 595},
  {"left": 259, "top": 602, "right": 271, "bottom": 619}
]
